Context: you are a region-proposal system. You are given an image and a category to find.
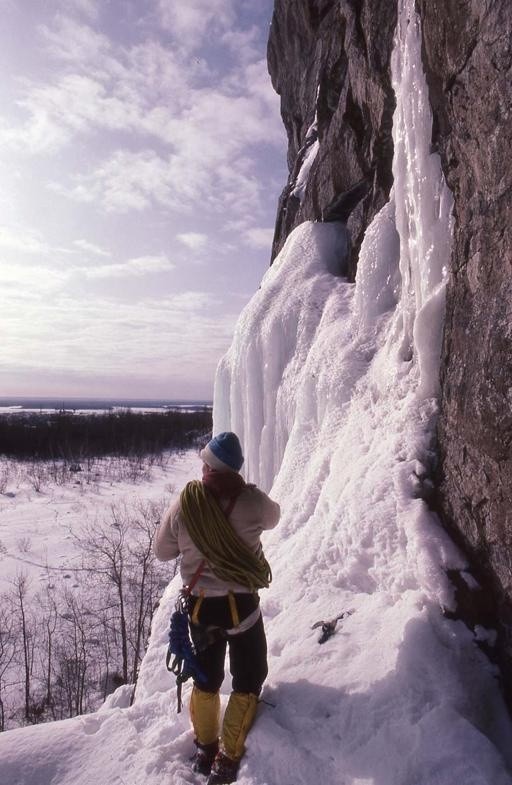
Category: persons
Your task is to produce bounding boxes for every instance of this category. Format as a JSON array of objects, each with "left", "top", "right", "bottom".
[{"left": 153, "top": 433, "right": 280, "bottom": 785}]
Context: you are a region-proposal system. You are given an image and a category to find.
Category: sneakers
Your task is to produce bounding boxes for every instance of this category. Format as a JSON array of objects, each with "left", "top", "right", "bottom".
[{"left": 191, "top": 747, "right": 240, "bottom": 785}]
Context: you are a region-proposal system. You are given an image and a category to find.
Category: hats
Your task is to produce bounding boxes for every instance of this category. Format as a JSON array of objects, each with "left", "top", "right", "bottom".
[{"left": 200, "top": 432, "right": 243, "bottom": 473}]
